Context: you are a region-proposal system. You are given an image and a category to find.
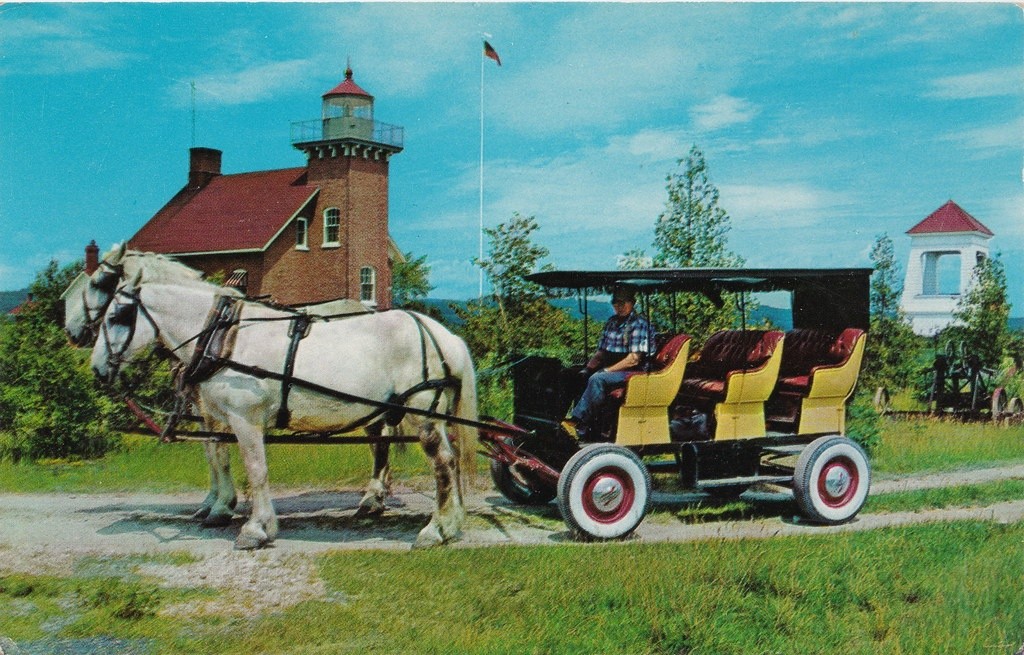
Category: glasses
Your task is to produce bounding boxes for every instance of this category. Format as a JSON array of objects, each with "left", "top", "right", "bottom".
[{"left": 610, "top": 299, "right": 632, "bottom": 306}]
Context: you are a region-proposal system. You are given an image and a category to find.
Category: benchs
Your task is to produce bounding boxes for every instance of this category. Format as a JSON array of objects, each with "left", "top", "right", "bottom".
[
  {"left": 671, "top": 330, "right": 786, "bottom": 439},
  {"left": 569, "top": 333, "right": 692, "bottom": 444},
  {"left": 764, "top": 328, "right": 868, "bottom": 434}
]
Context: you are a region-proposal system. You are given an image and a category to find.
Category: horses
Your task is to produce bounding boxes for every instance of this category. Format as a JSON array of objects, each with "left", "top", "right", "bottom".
[{"left": 64, "top": 239, "right": 480, "bottom": 549}]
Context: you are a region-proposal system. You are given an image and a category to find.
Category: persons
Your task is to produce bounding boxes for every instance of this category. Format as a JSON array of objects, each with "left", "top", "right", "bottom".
[{"left": 564, "top": 290, "right": 656, "bottom": 425}]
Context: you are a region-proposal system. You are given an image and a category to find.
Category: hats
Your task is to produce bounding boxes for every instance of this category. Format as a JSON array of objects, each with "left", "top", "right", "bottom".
[{"left": 610, "top": 283, "right": 637, "bottom": 305}]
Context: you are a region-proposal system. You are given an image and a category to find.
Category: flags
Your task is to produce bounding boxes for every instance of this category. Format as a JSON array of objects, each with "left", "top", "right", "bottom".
[{"left": 483, "top": 41, "right": 502, "bottom": 66}]
